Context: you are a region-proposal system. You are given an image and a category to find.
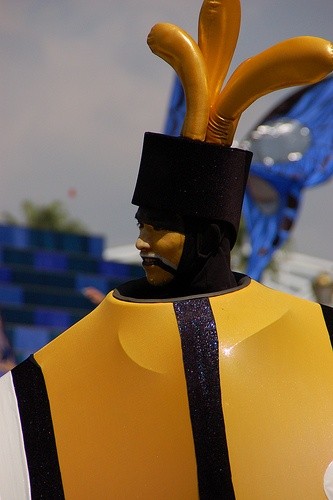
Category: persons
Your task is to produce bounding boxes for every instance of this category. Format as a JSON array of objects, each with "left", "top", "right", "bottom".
[{"left": 133, "top": 206, "right": 237, "bottom": 294}]
